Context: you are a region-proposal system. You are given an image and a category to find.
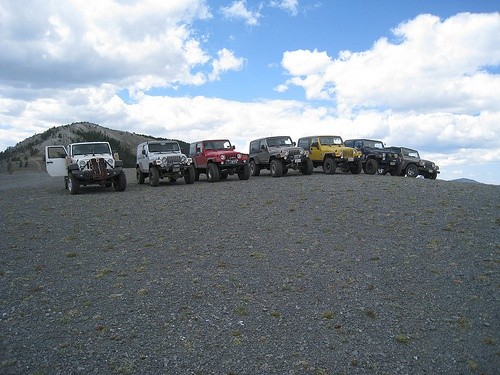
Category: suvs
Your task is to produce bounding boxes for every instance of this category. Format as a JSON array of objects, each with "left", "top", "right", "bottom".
[
  {"left": 189, "top": 138, "right": 250, "bottom": 182},
  {"left": 249, "top": 135, "right": 314, "bottom": 178},
  {"left": 45, "top": 141, "right": 127, "bottom": 195},
  {"left": 297, "top": 135, "right": 363, "bottom": 175},
  {"left": 385, "top": 146, "right": 440, "bottom": 180},
  {"left": 135, "top": 139, "right": 195, "bottom": 188},
  {"left": 343, "top": 138, "right": 399, "bottom": 175}
]
